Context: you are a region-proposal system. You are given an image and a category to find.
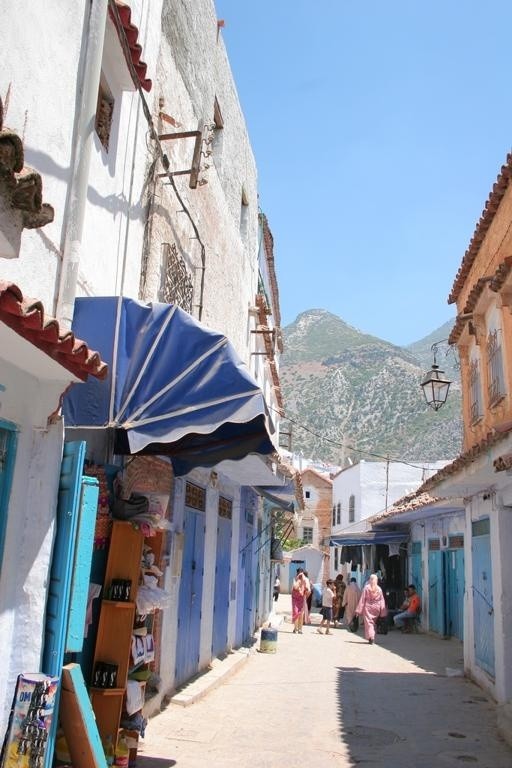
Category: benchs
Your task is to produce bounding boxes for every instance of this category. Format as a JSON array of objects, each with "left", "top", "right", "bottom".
[{"left": 402, "top": 608, "right": 421, "bottom": 633}]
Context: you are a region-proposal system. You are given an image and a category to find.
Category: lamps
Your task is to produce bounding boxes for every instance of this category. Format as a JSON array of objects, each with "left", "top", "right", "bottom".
[{"left": 419, "top": 339, "right": 453, "bottom": 410}]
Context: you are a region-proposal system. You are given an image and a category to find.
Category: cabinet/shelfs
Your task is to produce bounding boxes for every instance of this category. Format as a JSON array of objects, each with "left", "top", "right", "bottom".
[{"left": 86, "top": 518, "right": 170, "bottom": 768}]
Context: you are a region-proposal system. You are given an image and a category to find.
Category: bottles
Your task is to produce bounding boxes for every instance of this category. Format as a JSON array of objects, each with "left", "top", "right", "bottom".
[{"left": 115, "top": 734, "right": 129, "bottom": 768}]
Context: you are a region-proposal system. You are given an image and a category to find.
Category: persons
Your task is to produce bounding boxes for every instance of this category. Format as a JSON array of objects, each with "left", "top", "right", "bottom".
[
  {"left": 355, "top": 573, "right": 387, "bottom": 645},
  {"left": 304, "top": 570, "right": 420, "bottom": 635},
  {"left": 291, "top": 567, "right": 307, "bottom": 634},
  {"left": 273, "top": 575, "right": 280, "bottom": 601}
]
